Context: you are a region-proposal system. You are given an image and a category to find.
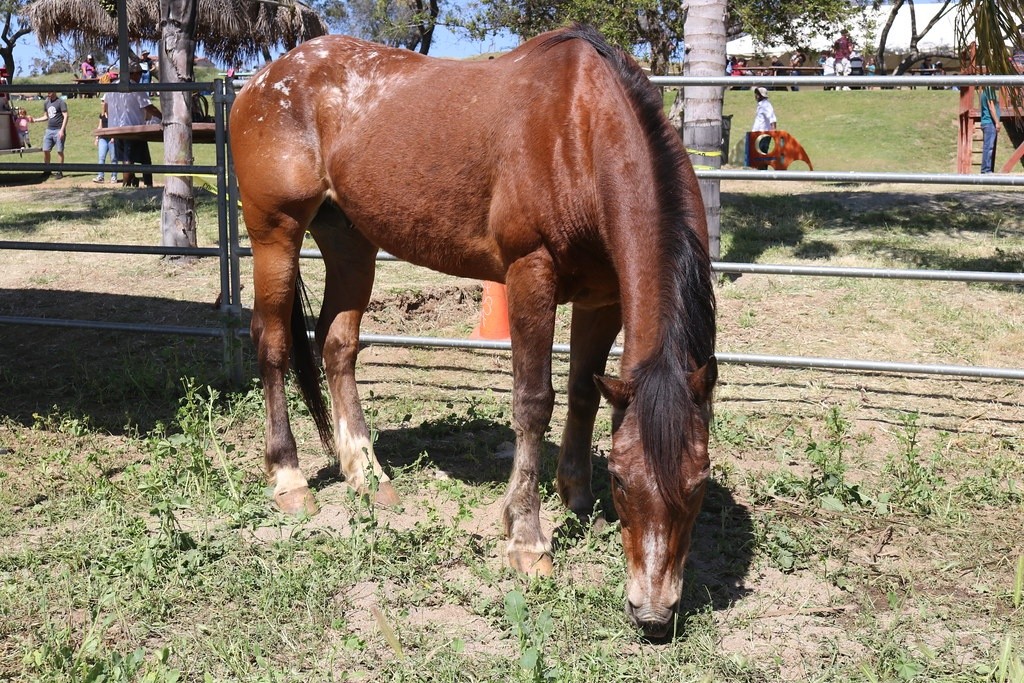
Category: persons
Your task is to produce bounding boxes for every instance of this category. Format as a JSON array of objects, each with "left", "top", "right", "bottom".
[
  {"left": 93, "top": 95, "right": 118, "bottom": 184},
  {"left": 771, "top": 56, "right": 788, "bottom": 91},
  {"left": 980, "top": 86, "right": 1001, "bottom": 174},
  {"left": 752, "top": 88, "right": 777, "bottom": 170},
  {"left": 15, "top": 108, "right": 35, "bottom": 148},
  {"left": 832, "top": 29, "right": 852, "bottom": 90},
  {"left": 866, "top": 58, "right": 875, "bottom": 90},
  {"left": 726, "top": 56, "right": 768, "bottom": 90},
  {"left": 32, "top": 92, "right": 68, "bottom": 179},
  {"left": 82, "top": 55, "right": 95, "bottom": 79},
  {"left": 228, "top": 66, "right": 236, "bottom": 77},
  {"left": 920, "top": 58, "right": 959, "bottom": 90},
  {"left": 138, "top": 50, "right": 156, "bottom": 96},
  {"left": 819, "top": 50, "right": 836, "bottom": 90},
  {"left": 104, "top": 62, "right": 162, "bottom": 187},
  {"left": 790, "top": 49, "right": 803, "bottom": 91}
]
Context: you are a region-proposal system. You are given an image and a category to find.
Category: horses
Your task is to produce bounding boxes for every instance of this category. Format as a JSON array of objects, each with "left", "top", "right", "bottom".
[{"left": 228, "top": 25, "right": 719, "bottom": 639}]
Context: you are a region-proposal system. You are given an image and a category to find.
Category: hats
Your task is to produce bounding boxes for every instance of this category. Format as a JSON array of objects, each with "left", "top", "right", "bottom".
[
  {"left": 142, "top": 50, "right": 150, "bottom": 55},
  {"left": 88, "top": 55, "right": 93, "bottom": 59},
  {"left": 758, "top": 87, "right": 769, "bottom": 98},
  {"left": 129, "top": 62, "right": 147, "bottom": 74}
]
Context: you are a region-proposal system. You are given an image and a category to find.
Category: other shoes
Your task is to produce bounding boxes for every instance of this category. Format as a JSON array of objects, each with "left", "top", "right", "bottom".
[
  {"left": 111, "top": 175, "right": 118, "bottom": 182},
  {"left": 40, "top": 172, "right": 50, "bottom": 178},
  {"left": 842, "top": 86, "right": 854, "bottom": 91},
  {"left": 93, "top": 177, "right": 105, "bottom": 182},
  {"left": 836, "top": 86, "right": 842, "bottom": 91},
  {"left": 56, "top": 171, "right": 64, "bottom": 179}
]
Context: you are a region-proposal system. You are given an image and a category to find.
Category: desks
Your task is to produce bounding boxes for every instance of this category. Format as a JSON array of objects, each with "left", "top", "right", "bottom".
[
  {"left": 90, "top": 122, "right": 226, "bottom": 187},
  {"left": 73, "top": 78, "right": 119, "bottom": 98}
]
